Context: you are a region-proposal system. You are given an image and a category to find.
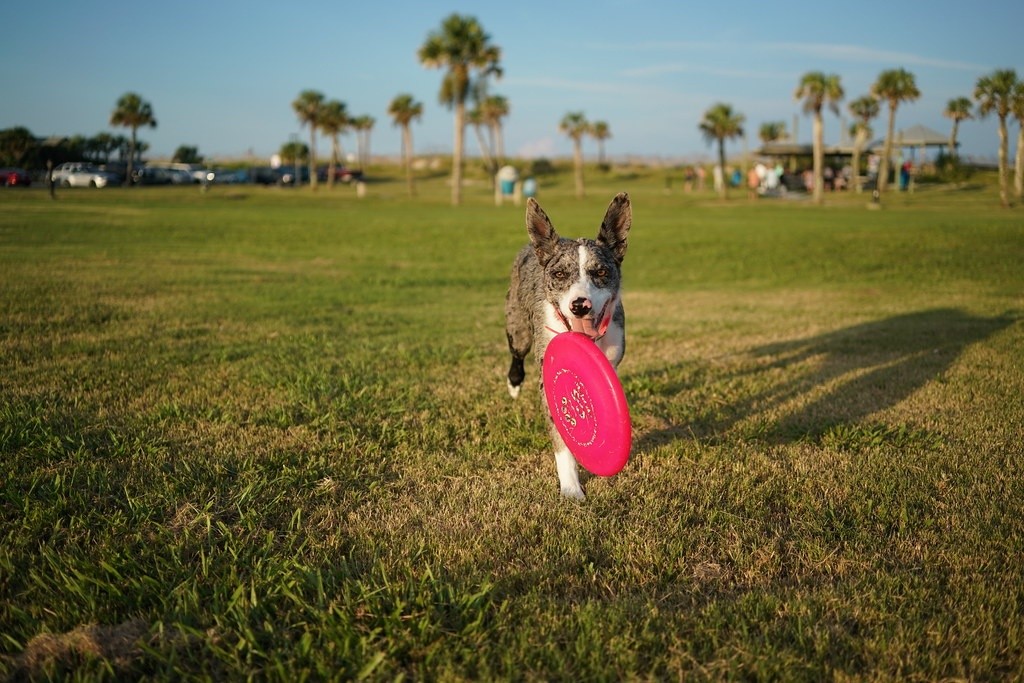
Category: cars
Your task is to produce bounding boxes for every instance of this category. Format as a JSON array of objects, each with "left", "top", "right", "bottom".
[
  {"left": 0, "top": 167, "right": 34, "bottom": 188},
  {"left": 46, "top": 162, "right": 122, "bottom": 189},
  {"left": 137, "top": 161, "right": 364, "bottom": 186}
]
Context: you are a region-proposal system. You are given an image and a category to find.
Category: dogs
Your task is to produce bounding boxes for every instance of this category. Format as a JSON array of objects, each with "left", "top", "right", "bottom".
[{"left": 504, "top": 191, "right": 631, "bottom": 505}]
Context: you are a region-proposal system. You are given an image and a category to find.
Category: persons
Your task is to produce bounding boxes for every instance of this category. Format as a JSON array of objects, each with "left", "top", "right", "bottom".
[
  {"left": 45, "top": 161, "right": 58, "bottom": 200},
  {"left": 684, "top": 159, "right": 913, "bottom": 199}
]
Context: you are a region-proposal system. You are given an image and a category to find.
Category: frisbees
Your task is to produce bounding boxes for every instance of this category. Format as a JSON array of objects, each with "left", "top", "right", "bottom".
[{"left": 542, "top": 331, "right": 634, "bottom": 477}]
